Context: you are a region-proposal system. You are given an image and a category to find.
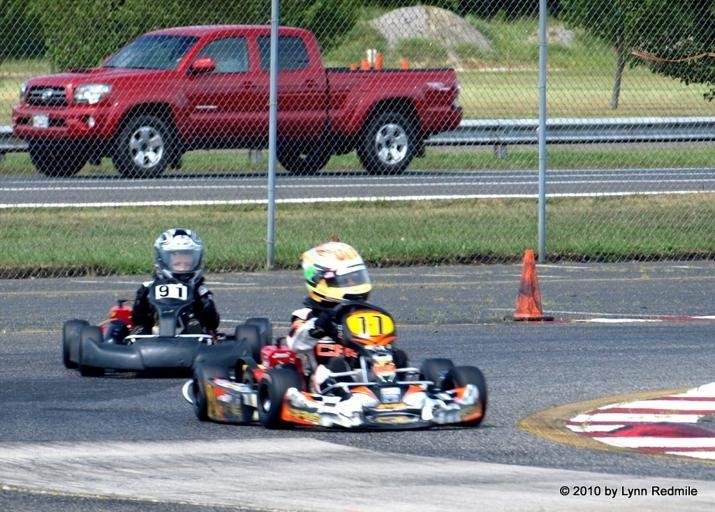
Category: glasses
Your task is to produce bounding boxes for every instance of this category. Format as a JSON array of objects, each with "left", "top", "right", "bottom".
[{"left": 327, "top": 273, "right": 361, "bottom": 285}]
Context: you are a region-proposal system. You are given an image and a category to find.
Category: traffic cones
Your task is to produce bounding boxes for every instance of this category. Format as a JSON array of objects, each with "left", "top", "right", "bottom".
[{"left": 513, "top": 248, "right": 554, "bottom": 322}]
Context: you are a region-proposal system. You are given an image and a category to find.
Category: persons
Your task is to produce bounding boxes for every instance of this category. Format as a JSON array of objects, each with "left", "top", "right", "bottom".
[
  {"left": 284, "top": 240, "right": 407, "bottom": 397},
  {"left": 132, "top": 227, "right": 219, "bottom": 334}
]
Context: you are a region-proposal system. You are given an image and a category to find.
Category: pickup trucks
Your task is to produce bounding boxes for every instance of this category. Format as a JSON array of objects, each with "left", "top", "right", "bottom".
[{"left": 10, "top": 24, "right": 465, "bottom": 179}]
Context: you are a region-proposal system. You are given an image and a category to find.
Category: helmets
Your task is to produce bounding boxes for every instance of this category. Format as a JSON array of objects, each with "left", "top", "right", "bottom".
[
  {"left": 302, "top": 241, "right": 372, "bottom": 309},
  {"left": 153, "top": 228, "right": 204, "bottom": 287}
]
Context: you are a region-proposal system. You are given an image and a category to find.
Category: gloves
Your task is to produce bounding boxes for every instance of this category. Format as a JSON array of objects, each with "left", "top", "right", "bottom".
[{"left": 315, "top": 310, "right": 336, "bottom": 328}]
